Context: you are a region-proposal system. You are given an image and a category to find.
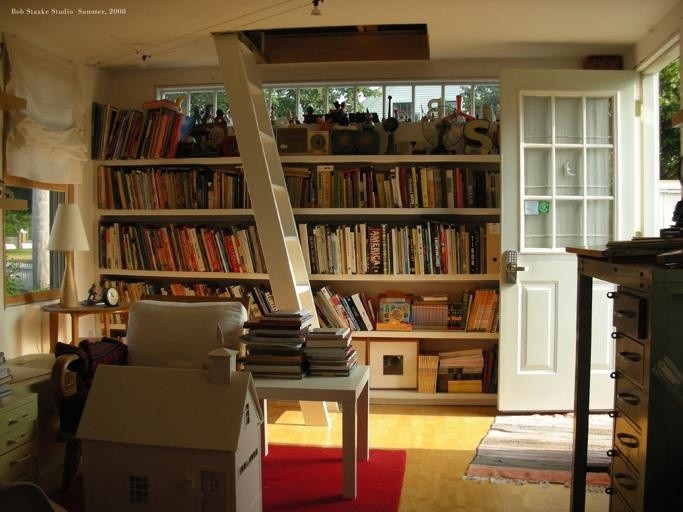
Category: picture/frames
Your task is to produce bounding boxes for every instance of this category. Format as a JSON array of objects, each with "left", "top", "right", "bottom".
[{"left": 352, "top": 335, "right": 420, "bottom": 391}]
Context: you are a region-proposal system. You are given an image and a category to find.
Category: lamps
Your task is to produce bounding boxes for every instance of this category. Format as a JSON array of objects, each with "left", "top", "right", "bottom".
[{"left": 46, "top": 202, "right": 90, "bottom": 308}]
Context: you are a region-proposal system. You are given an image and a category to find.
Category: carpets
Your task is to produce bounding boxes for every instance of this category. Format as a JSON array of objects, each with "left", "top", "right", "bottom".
[
  {"left": 49, "top": 443, "right": 406, "bottom": 511},
  {"left": 461, "top": 410, "right": 613, "bottom": 490}
]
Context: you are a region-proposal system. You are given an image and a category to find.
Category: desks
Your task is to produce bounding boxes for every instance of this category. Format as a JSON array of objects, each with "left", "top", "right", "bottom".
[
  {"left": 563, "top": 236, "right": 682, "bottom": 511},
  {"left": 246, "top": 364, "right": 371, "bottom": 499},
  {"left": 39, "top": 302, "right": 130, "bottom": 355}
]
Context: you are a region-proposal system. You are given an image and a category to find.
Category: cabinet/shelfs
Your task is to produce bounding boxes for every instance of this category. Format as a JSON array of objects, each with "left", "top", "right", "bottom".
[
  {"left": 0, "top": 390, "right": 40, "bottom": 485},
  {"left": 94, "top": 153, "right": 500, "bottom": 406}
]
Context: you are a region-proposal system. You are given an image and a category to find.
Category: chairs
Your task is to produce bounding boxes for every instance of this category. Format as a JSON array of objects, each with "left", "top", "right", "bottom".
[{"left": 50, "top": 293, "right": 249, "bottom": 494}]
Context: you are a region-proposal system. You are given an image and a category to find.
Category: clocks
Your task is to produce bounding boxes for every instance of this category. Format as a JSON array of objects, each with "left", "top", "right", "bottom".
[{"left": 102, "top": 286, "right": 118, "bottom": 306}]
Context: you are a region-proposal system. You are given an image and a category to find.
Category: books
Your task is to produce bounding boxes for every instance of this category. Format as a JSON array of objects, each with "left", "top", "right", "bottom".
[
  {"left": 90, "top": 101, "right": 192, "bottom": 162},
  {"left": 94, "top": 164, "right": 498, "bottom": 393},
  {"left": 566, "top": 234, "right": 683, "bottom": 269}
]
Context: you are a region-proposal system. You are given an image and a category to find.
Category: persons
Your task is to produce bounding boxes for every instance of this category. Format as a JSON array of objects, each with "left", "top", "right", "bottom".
[{"left": 88, "top": 283, "right": 100, "bottom": 302}]
